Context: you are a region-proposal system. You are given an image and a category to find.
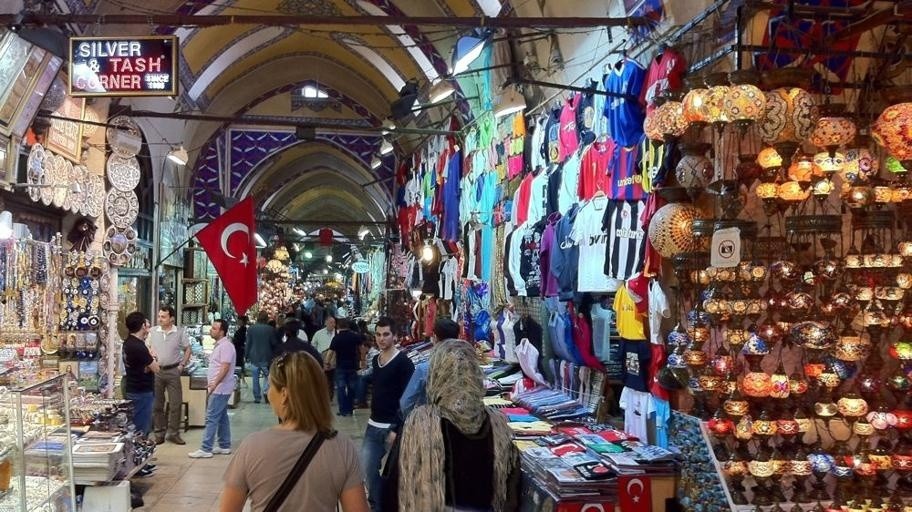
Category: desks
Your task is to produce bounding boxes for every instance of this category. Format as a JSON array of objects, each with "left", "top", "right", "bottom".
[{"left": 519, "top": 465, "right": 680, "bottom": 512}]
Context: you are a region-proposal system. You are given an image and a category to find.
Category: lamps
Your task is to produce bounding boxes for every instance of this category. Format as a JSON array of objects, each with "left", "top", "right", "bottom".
[
  {"left": 370, "top": 73, "right": 455, "bottom": 170},
  {"left": 325, "top": 246, "right": 332, "bottom": 263},
  {"left": 422, "top": 238, "right": 434, "bottom": 263},
  {"left": 166, "top": 144, "right": 189, "bottom": 166},
  {"left": 303, "top": 248, "right": 313, "bottom": 259},
  {"left": 491, "top": 77, "right": 639, "bottom": 118},
  {"left": 449, "top": 29, "right": 492, "bottom": 76}
]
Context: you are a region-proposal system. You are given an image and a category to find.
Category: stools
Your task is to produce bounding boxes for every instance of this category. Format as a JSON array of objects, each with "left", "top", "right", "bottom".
[
  {"left": 81, "top": 480, "right": 133, "bottom": 512},
  {"left": 166, "top": 400, "right": 189, "bottom": 432}
]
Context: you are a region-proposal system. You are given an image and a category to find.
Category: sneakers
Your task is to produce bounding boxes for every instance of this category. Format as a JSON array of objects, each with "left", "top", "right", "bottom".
[
  {"left": 213, "top": 447, "right": 231, "bottom": 454},
  {"left": 133, "top": 464, "right": 156, "bottom": 477},
  {"left": 188, "top": 450, "right": 212, "bottom": 458},
  {"left": 151, "top": 437, "right": 164, "bottom": 444},
  {"left": 353, "top": 401, "right": 368, "bottom": 408},
  {"left": 166, "top": 433, "right": 186, "bottom": 444}
]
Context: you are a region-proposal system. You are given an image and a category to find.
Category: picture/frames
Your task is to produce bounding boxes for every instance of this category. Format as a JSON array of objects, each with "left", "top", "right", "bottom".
[{"left": 42, "top": 69, "right": 87, "bottom": 164}]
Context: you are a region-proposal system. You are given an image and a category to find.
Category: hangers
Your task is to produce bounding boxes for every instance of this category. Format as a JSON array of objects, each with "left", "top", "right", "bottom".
[{"left": 527, "top": 35, "right": 667, "bottom": 217}]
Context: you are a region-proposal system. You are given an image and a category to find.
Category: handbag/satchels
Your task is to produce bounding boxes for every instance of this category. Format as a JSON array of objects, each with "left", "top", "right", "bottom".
[{"left": 321, "top": 348, "right": 337, "bottom": 371}]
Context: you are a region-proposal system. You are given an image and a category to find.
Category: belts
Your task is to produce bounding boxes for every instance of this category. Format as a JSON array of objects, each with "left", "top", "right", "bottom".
[{"left": 160, "top": 362, "right": 178, "bottom": 370}]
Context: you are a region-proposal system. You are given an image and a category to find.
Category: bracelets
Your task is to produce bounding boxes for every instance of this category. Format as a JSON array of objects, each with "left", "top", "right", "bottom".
[{"left": 179, "top": 363, "right": 185, "bottom": 367}]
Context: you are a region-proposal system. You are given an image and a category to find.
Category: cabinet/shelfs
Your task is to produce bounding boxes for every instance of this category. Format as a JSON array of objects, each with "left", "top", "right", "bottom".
[{"left": 0, "top": 369, "right": 78, "bottom": 512}]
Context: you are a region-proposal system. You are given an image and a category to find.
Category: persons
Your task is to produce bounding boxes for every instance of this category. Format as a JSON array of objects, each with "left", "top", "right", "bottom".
[
  {"left": 381, "top": 339, "right": 519, "bottom": 512},
  {"left": 398, "top": 319, "right": 460, "bottom": 419},
  {"left": 146, "top": 306, "right": 192, "bottom": 445},
  {"left": 63, "top": 365, "right": 78, "bottom": 380},
  {"left": 121, "top": 319, "right": 150, "bottom": 400},
  {"left": 208, "top": 289, "right": 368, "bottom": 416},
  {"left": 188, "top": 320, "right": 232, "bottom": 457},
  {"left": 219, "top": 351, "right": 369, "bottom": 511},
  {"left": 361, "top": 317, "right": 416, "bottom": 511},
  {"left": 122, "top": 311, "right": 157, "bottom": 477}
]
